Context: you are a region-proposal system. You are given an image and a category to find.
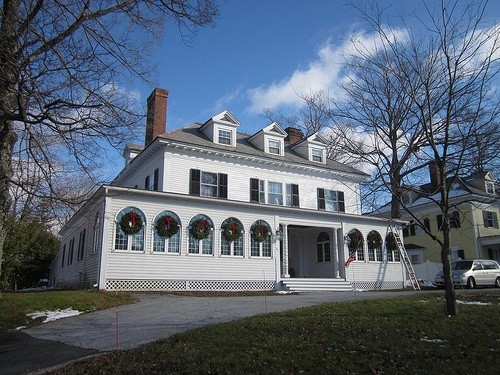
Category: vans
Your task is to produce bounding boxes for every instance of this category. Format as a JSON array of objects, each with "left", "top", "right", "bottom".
[{"left": 435, "top": 259, "right": 500, "bottom": 288}]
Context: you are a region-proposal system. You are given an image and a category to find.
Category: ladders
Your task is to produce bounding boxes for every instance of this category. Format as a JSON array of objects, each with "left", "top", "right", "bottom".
[{"left": 389, "top": 224, "right": 423, "bottom": 292}]
